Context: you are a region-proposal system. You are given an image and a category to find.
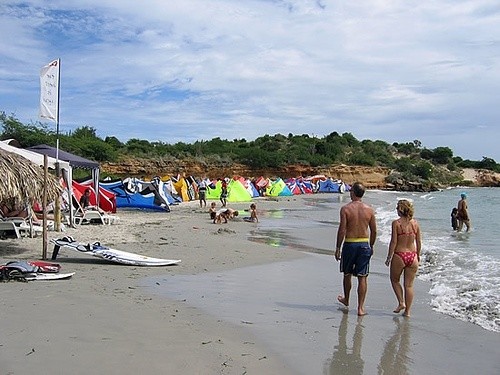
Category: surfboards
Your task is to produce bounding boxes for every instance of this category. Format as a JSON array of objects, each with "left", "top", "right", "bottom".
[
  {"left": 22, "top": 271, "right": 76, "bottom": 280},
  {"left": 49, "top": 236, "right": 181, "bottom": 266}
]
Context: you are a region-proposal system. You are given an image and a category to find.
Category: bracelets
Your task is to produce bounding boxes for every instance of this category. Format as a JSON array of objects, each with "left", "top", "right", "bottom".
[{"left": 387, "top": 256, "right": 391, "bottom": 259}]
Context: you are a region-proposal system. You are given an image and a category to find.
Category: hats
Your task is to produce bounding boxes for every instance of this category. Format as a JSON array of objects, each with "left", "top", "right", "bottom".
[{"left": 461, "top": 193, "right": 467, "bottom": 198}]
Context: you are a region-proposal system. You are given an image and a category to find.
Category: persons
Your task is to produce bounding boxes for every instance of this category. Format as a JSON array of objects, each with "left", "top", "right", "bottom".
[
  {"left": 451, "top": 192, "right": 471, "bottom": 233},
  {"left": 243, "top": 203, "right": 260, "bottom": 223},
  {"left": 335, "top": 182, "right": 377, "bottom": 316},
  {"left": 220, "top": 180, "right": 228, "bottom": 208},
  {"left": 197, "top": 177, "right": 210, "bottom": 208},
  {"left": 385, "top": 200, "right": 422, "bottom": 317},
  {"left": 209, "top": 202, "right": 238, "bottom": 224},
  {"left": 79, "top": 188, "right": 92, "bottom": 209}
]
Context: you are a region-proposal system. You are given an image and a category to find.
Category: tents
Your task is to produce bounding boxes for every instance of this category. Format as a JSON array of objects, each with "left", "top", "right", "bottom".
[
  {"left": 0, "top": 139, "right": 117, "bottom": 238},
  {"left": 190, "top": 173, "right": 353, "bottom": 202},
  {"left": 73, "top": 171, "right": 199, "bottom": 213}
]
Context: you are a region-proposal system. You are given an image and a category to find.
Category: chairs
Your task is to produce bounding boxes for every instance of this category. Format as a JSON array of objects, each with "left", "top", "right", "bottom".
[{"left": 0, "top": 206, "right": 122, "bottom": 239}]
